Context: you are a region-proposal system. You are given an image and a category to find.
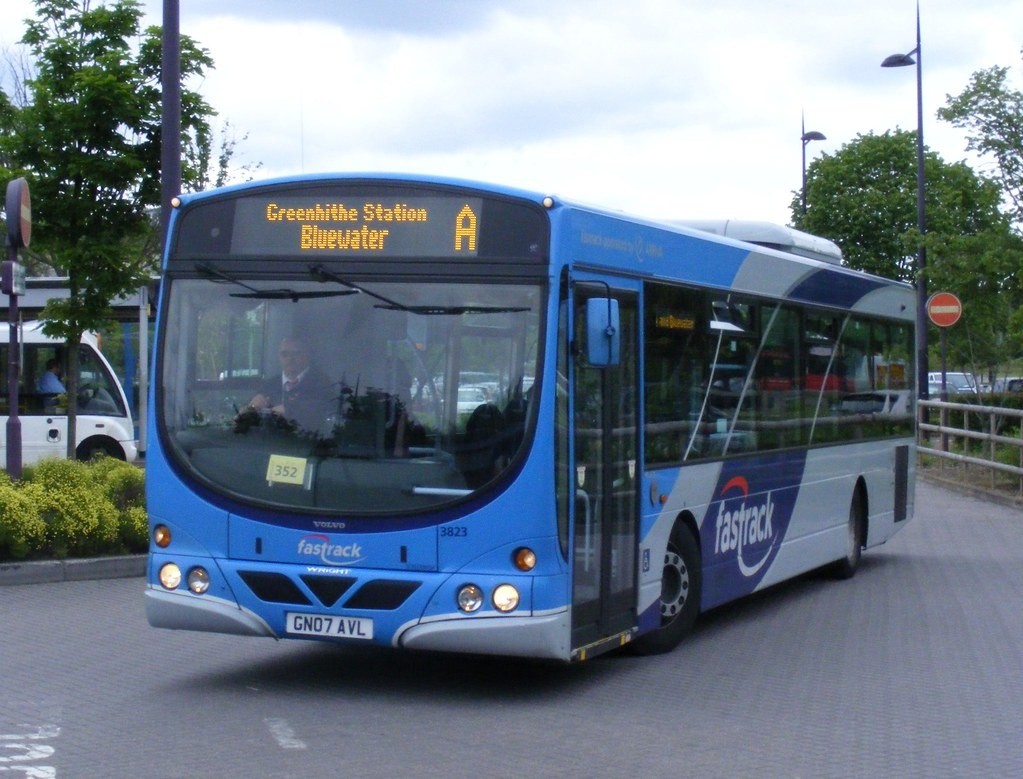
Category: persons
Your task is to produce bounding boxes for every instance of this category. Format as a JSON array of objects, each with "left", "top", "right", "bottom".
[
  {"left": 630, "top": 361, "right": 663, "bottom": 396},
  {"left": 457, "top": 395, "right": 528, "bottom": 492},
  {"left": 388, "top": 355, "right": 413, "bottom": 412},
  {"left": 39, "top": 358, "right": 67, "bottom": 393},
  {"left": 248, "top": 332, "right": 330, "bottom": 431}
]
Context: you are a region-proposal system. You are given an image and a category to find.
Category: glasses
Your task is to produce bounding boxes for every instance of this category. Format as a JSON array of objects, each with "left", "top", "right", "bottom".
[{"left": 278, "top": 349, "right": 305, "bottom": 358}]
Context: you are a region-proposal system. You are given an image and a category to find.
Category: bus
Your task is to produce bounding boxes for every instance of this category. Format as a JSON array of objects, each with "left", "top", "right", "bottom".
[
  {"left": 142, "top": 169, "right": 923, "bottom": 665},
  {"left": 0, "top": 318, "right": 137, "bottom": 476}
]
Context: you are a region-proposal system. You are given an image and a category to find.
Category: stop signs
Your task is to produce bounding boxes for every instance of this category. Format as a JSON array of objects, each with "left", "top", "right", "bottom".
[
  {"left": 928, "top": 293, "right": 962, "bottom": 327},
  {"left": 20, "top": 177, "right": 32, "bottom": 247}
]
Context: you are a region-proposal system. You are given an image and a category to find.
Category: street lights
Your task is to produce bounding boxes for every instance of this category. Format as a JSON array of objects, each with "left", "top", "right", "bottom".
[
  {"left": 801, "top": 111, "right": 827, "bottom": 231},
  {"left": 880, "top": 0, "right": 931, "bottom": 467}
]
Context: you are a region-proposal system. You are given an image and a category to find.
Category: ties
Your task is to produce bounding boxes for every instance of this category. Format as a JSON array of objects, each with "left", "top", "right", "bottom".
[{"left": 287, "top": 382, "right": 296, "bottom": 401}]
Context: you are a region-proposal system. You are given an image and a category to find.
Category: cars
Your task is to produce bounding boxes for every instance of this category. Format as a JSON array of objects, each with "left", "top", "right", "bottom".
[
  {"left": 407, "top": 366, "right": 536, "bottom": 421},
  {"left": 927, "top": 370, "right": 1023, "bottom": 396},
  {"left": 746, "top": 341, "right": 910, "bottom": 415}
]
